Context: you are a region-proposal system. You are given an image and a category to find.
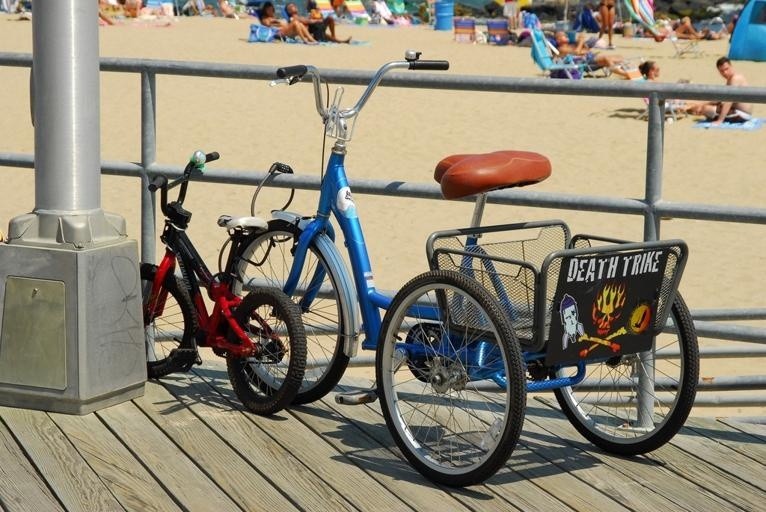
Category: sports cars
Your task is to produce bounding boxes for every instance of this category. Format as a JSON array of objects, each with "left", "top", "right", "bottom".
[{"left": 222, "top": 50, "right": 702, "bottom": 490}]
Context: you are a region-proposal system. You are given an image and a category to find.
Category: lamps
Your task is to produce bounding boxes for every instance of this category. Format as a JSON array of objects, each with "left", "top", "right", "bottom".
[
  {"left": 453, "top": 17, "right": 511, "bottom": 45},
  {"left": 530, "top": 29, "right": 588, "bottom": 81},
  {"left": 313, "top": 0, "right": 422, "bottom": 25},
  {"left": 250, "top": 4, "right": 295, "bottom": 43},
  {"left": 621, "top": 57, "right": 700, "bottom": 124}
]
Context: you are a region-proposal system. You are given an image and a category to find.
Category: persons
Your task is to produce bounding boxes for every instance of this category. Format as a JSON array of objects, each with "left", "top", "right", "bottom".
[
  {"left": 633, "top": 55, "right": 751, "bottom": 125},
  {"left": 558, "top": 26, "right": 630, "bottom": 80},
  {"left": 585, "top": 0, "right": 739, "bottom": 48},
  {"left": 98, "top": 0, "right": 243, "bottom": 26},
  {"left": 261, "top": 0, "right": 352, "bottom": 44}
]
